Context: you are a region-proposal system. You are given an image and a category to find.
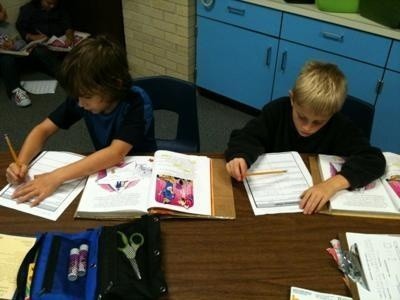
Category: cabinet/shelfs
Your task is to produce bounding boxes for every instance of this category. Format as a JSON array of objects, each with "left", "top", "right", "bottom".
[
  {"left": 373, "top": 41, "right": 400, "bottom": 155},
  {"left": 195, "top": 0, "right": 393, "bottom": 126}
]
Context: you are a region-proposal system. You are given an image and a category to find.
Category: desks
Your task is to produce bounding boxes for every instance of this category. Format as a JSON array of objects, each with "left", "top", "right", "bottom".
[{"left": 0, "top": 149, "right": 400, "bottom": 300}]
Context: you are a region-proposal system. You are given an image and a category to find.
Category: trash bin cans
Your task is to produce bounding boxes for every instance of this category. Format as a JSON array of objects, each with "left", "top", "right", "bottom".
[{"left": 103, "top": 11, "right": 124, "bottom": 50}]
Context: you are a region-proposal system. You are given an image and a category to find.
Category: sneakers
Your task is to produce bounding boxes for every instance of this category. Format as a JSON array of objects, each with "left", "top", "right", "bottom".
[{"left": 7, "top": 86, "right": 33, "bottom": 108}]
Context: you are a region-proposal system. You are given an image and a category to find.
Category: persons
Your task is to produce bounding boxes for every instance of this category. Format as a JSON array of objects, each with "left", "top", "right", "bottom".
[
  {"left": 16, "top": 1, "right": 92, "bottom": 89},
  {"left": 224, "top": 61, "right": 386, "bottom": 216},
  {"left": 0, "top": 4, "right": 33, "bottom": 108},
  {"left": 5, "top": 33, "right": 156, "bottom": 209}
]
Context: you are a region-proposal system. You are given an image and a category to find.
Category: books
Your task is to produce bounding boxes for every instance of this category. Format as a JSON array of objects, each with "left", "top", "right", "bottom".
[
  {"left": 73, "top": 150, "right": 237, "bottom": 221},
  {"left": 310, "top": 151, "right": 400, "bottom": 221}
]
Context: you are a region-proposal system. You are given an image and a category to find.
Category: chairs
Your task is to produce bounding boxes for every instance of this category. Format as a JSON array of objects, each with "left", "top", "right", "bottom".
[
  {"left": 134, "top": 76, "right": 202, "bottom": 154},
  {"left": 344, "top": 96, "right": 375, "bottom": 143}
]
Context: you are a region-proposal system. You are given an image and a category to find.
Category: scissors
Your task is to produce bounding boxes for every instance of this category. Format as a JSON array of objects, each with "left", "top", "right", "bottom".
[{"left": 107, "top": 230, "right": 145, "bottom": 280}]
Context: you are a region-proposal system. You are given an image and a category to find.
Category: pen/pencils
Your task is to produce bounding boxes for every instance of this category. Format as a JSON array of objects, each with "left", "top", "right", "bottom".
[
  {"left": 3, "top": 133, "right": 27, "bottom": 183},
  {"left": 246, "top": 171, "right": 286, "bottom": 176}
]
